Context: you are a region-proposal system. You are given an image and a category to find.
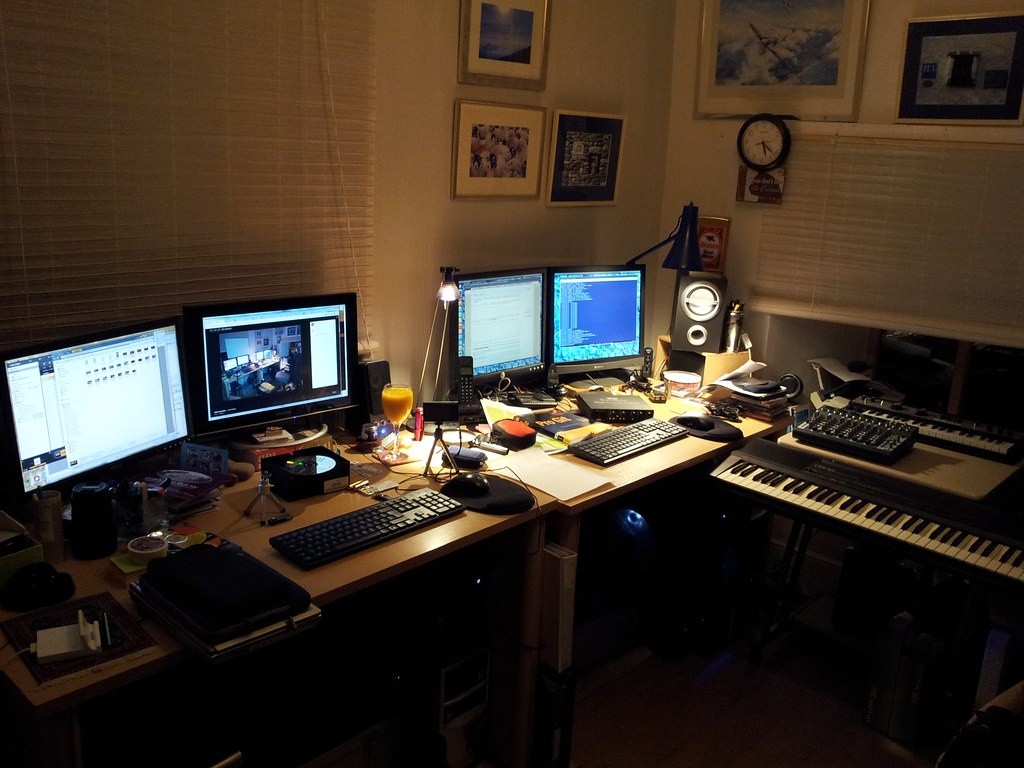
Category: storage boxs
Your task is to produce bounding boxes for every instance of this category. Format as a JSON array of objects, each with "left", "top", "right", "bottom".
[
  {"left": 652, "top": 335, "right": 751, "bottom": 390},
  {"left": 0, "top": 510, "right": 43, "bottom": 570}
]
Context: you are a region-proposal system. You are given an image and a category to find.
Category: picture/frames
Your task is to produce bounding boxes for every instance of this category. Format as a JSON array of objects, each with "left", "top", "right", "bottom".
[
  {"left": 457, "top": 0, "right": 552, "bottom": 93},
  {"left": 545, "top": 109, "right": 627, "bottom": 207},
  {"left": 697, "top": 215, "right": 731, "bottom": 272},
  {"left": 693, "top": 0, "right": 872, "bottom": 122},
  {"left": 449, "top": 98, "right": 548, "bottom": 200},
  {"left": 179, "top": 441, "right": 230, "bottom": 475},
  {"left": 893, "top": 10, "right": 1024, "bottom": 125}
]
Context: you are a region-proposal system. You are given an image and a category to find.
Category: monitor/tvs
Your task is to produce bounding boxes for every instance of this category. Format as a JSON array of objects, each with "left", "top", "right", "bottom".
[
  {"left": 448, "top": 263, "right": 645, "bottom": 408},
  {"left": 0, "top": 291, "right": 361, "bottom": 502}
]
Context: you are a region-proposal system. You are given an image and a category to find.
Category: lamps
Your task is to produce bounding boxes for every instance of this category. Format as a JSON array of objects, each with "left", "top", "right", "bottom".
[
  {"left": 411, "top": 265, "right": 463, "bottom": 415},
  {"left": 626, "top": 202, "right": 705, "bottom": 274}
]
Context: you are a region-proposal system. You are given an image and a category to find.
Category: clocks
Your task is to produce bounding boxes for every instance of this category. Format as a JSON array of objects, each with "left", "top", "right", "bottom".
[{"left": 737, "top": 112, "right": 792, "bottom": 172}]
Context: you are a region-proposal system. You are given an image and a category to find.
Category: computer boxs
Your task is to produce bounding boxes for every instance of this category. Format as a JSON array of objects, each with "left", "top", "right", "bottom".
[
  {"left": 626, "top": 498, "right": 775, "bottom": 659},
  {"left": 381, "top": 627, "right": 497, "bottom": 768}
]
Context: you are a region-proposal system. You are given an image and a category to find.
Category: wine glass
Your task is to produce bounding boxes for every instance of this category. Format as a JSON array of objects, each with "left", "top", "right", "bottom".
[{"left": 381, "top": 383, "right": 413, "bottom": 461}]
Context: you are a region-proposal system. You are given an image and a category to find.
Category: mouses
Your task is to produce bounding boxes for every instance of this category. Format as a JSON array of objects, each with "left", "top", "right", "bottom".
[
  {"left": 440, "top": 473, "right": 490, "bottom": 498},
  {"left": 677, "top": 412, "right": 715, "bottom": 431}
]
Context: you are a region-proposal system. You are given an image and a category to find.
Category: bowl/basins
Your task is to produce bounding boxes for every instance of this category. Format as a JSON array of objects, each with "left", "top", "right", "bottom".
[{"left": 662, "top": 370, "right": 702, "bottom": 395}]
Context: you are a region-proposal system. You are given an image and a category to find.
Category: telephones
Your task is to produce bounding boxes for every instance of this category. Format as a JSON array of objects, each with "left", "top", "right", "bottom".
[
  {"left": 458, "top": 353, "right": 473, "bottom": 405},
  {"left": 643, "top": 347, "right": 654, "bottom": 382}
]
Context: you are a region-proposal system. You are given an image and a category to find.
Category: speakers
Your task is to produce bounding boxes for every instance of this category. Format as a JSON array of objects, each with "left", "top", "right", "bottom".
[
  {"left": 345, "top": 359, "right": 391, "bottom": 436},
  {"left": 670, "top": 268, "right": 728, "bottom": 353}
]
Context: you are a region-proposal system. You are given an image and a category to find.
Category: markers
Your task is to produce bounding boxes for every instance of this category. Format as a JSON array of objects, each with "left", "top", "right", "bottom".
[{"left": 133, "top": 476, "right": 172, "bottom": 520}]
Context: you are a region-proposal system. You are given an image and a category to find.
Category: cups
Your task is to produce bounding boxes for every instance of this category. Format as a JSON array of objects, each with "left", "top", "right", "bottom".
[
  {"left": 32, "top": 490, "right": 64, "bottom": 549},
  {"left": 137, "top": 492, "right": 169, "bottom": 533}
]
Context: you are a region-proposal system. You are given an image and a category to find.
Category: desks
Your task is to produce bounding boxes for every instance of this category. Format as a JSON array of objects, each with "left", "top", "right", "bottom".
[{"left": 0, "top": 367, "right": 798, "bottom": 768}]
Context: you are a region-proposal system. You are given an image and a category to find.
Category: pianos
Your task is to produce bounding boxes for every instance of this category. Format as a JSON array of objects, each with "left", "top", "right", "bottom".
[{"left": 707, "top": 394, "right": 1024, "bottom": 721}]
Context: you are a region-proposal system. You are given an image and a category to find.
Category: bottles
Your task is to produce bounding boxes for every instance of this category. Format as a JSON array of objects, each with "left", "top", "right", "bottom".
[
  {"left": 70, "top": 480, "right": 118, "bottom": 561},
  {"left": 720, "top": 308, "right": 743, "bottom": 354}
]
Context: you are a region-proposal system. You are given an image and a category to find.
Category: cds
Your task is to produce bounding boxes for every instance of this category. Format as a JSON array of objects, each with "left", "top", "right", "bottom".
[
  {"left": 284, "top": 455, "right": 336, "bottom": 476},
  {"left": 148, "top": 523, "right": 207, "bottom": 551}
]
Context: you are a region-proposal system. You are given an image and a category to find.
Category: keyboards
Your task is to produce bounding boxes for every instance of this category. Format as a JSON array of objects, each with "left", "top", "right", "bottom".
[
  {"left": 568, "top": 417, "right": 689, "bottom": 467},
  {"left": 269, "top": 488, "right": 466, "bottom": 570}
]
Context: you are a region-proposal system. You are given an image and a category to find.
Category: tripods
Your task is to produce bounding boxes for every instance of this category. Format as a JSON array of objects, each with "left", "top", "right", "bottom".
[
  {"left": 422, "top": 422, "right": 459, "bottom": 478},
  {"left": 243, "top": 471, "right": 286, "bottom": 527}
]
